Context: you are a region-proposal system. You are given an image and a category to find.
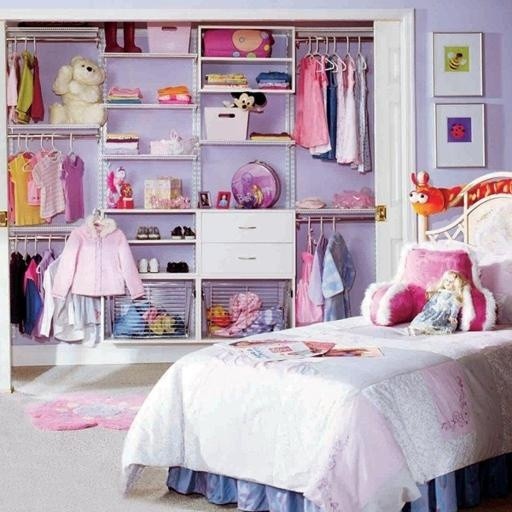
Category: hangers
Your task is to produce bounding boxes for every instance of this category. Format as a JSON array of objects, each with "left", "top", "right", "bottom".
[
  {"left": 11, "top": 234, "right": 67, "bottom": 275},
  {"left": 92, "top": 208, "right": 104, "bottom": 229},
  {"left": 295, "top": 35, "right": 368, "bottom": 73},
  {"left": 14, "top": 36, "right": 36, "bottom": 53},
  {"left": 8, "top": 133, "right": 77, "bottom": 172},
  {"left": 306, "top": 215, "right": 336, "bottom": 256}
]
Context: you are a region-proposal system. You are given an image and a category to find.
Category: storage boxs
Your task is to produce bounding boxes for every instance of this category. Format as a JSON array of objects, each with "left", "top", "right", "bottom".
[
  {"left": 147, "top": 22, "right": 192, "bottom": 53},
  {"left": 204, "top": 107, "right": 250, "bottom": 140}
]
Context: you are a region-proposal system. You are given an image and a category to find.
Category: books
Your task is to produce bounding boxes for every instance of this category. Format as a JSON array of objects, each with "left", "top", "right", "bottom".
[{"left": 228, "top": 336, "right": 385, "bottom": 364}]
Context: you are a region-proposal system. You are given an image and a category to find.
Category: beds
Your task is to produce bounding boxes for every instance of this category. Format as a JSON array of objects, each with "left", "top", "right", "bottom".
[{"left": 166, "top": 170, "right": 512, "bottom": 512}]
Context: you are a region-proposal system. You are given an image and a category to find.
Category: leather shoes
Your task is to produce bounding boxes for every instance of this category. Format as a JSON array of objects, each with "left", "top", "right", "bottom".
[{"left": 167, "top": 263, "right": 188, "bottom": 273}]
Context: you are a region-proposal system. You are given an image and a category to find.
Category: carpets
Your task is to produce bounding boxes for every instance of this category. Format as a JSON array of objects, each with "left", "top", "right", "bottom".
[{"left": 26, "top": 393, "right": 145, "bottom": 431}]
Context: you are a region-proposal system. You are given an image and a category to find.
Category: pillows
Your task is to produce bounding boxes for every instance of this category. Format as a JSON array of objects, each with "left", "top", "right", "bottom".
[{"left": 361, "top": 240, "right": 497, "bottom": 331}]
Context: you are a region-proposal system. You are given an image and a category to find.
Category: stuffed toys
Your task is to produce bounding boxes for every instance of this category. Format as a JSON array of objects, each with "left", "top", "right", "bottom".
[
  {"left": 407, "top": 173, "right": 512, "bottom": 216},
  {"left": 219, "top": 91, "right": 266, "bottom": 118},
  {"left": 47, "top": 55, "right": 108, "bottom": 126}
]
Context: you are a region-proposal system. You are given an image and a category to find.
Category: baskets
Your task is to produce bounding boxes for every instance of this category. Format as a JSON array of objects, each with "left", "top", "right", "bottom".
[
  {"left": 109, "top": 282, "right": 194, "bottom": 339},
  {"left": 202, "top": 282, "right": 289, "bottom": 338}
]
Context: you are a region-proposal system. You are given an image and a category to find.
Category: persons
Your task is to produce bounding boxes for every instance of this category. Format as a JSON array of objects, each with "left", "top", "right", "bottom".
[
  {"left": 220, "top": 194, "right": 228, "bottom": 207},
  {"left": 202, "top": 195, "right": 209, "bottom": 205},
  {"left": 403, "top": 269, "right": 471, "bottom": 337}
]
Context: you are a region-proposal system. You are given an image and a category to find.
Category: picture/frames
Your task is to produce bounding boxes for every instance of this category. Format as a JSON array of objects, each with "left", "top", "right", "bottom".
[
  {"left": 432, "top": 32, "right": 484, "bottom": 97},
  {"left": 434, "top": 102, "right": 486, "bottom": 168}
]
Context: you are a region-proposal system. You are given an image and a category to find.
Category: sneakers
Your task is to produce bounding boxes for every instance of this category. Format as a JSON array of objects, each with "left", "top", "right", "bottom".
[
  {"left": 138, "top": 258, "right": 158, "bottom": 273},
  {"left": 172, "top": 226, "right": 195, "bottom": 240},
  {"left": 137, "top": 227, "right": 159, "bottom": 240}
]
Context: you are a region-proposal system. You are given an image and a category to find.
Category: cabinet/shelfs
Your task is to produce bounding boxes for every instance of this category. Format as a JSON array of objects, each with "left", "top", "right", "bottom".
[{"left": 0, "top": 19, "right": 375, "bottom": 366}]
